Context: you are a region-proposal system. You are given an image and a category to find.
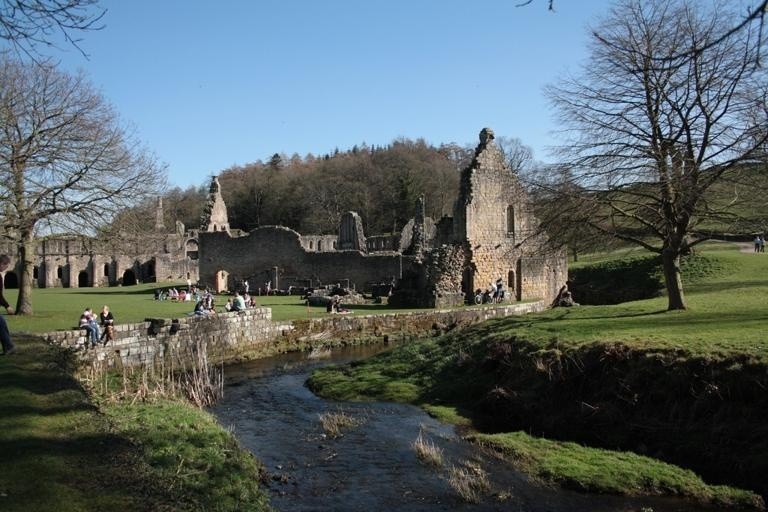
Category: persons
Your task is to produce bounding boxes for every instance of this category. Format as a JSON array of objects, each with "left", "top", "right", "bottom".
[
  {"left": 79, "top": 307, "right": 103, "bottom": 346},
  {"left": 265, "top": 281, "right": 271, "bottom": 296},
  {"left": 0, "top": 255, "right": 17, "bottom": 356},
  {"left": 242, "top": 280, "right": 249, "bottom": 293},
  {"left": 760, "top": 237, "right": 766, "bottom": 253},
  {"left": 753, "top": 236, "right": 761, "bottom": 253},
  {"left": 99, "top": 304, "right": 114, "bottom": 340},
  {"left": 462, "top": 277, "right": 504, "bottom": 305},
  {"left": 151, "top": 283, "right": 254, "bottom": 314}
]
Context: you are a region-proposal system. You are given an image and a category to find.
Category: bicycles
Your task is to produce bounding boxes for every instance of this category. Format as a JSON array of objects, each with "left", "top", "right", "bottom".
[{"left": 474, "top": 290, "right": 494, "bottom": 305}]
